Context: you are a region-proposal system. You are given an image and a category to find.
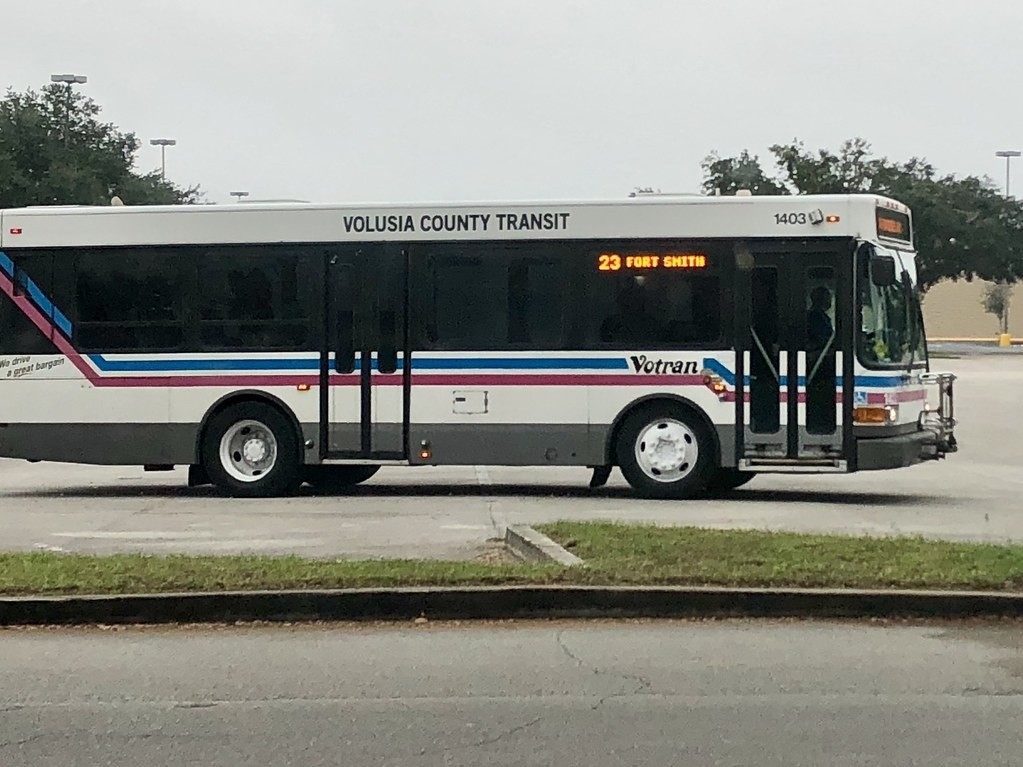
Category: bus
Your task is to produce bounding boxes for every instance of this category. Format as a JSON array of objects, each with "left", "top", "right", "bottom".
[{"left": 0, "top": 189, "right": 955, "bottom": 503}]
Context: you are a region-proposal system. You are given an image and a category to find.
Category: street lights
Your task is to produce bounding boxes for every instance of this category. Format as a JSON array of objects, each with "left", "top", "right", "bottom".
[
  {"left": 51, "top": 73, "right": 87, "bottom": 150},
  {"left": 995, "top": 150, "right": 1022, "bottom": 335},
  {"left": 150, "top": 139, "right": 175, "bottom": 182},
  {"left": 230, "top": 192, "right": 250, "bottom": 200}
]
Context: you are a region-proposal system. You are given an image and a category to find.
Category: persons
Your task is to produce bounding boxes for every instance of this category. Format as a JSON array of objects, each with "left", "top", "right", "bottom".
[
  {"left": 807, "top": 286, "right": 834, "bottom": 345},
  {"left": 839, "top": 297, "right": 875, "bottom": 351}
]
